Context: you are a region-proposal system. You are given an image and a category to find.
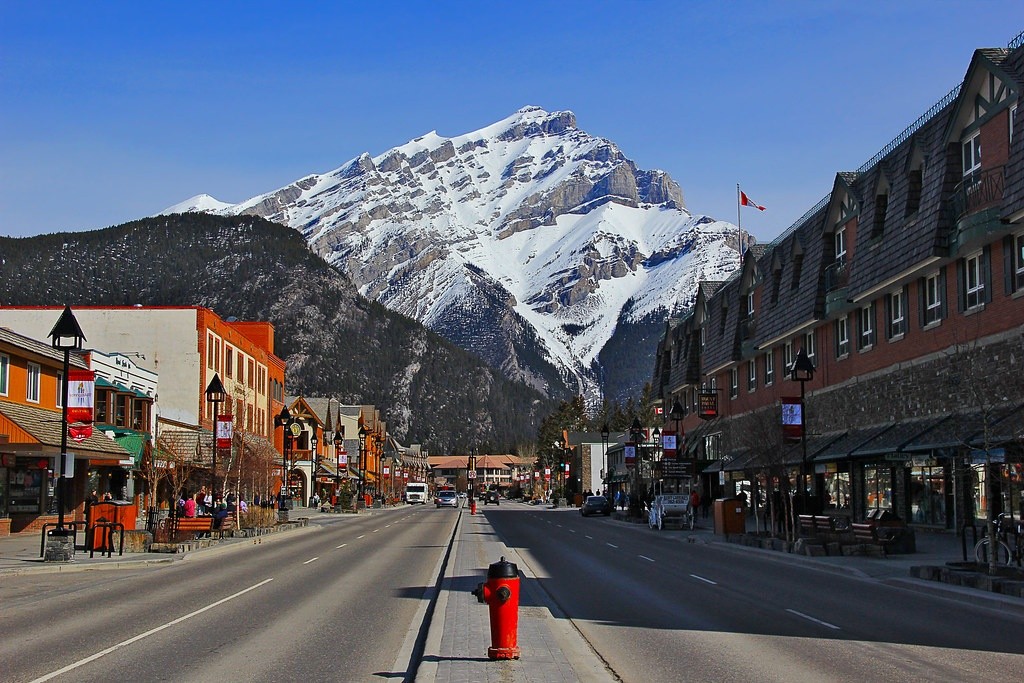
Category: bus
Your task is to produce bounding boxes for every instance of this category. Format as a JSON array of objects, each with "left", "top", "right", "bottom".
[{"left": 405, "top": 482, "right": 429, "bottom": 505}]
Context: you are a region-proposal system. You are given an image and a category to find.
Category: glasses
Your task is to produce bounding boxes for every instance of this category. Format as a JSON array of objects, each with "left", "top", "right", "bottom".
[{"left": 216, "top": 500, "right": 221, "bottom": 502}]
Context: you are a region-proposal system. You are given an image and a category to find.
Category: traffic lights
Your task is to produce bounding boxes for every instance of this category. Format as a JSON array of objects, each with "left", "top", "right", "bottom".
[{"left": 558, "top": 459, "right": 565, "bottom": 474}]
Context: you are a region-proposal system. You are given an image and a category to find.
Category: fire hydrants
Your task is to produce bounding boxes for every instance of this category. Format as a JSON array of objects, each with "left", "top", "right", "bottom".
[
  {"left": 469, "top": 500, "right": 476, "bottom": 515},
  {"left": 471, "top": 556, "right": 521, "bottom": 660}
]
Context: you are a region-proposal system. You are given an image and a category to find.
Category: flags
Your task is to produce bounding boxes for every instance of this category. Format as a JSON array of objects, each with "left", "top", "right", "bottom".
[
  {"left": 654, "top": 408, "right": 663, "bottom": 414},
  {"left": 741, "top": 191, "right": 766, "bottom": 211}
]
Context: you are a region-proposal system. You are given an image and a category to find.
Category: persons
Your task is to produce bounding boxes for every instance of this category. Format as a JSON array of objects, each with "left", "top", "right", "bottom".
[
  {"left": 582, "top": 489, "right": 594, "bottom": 503},
  {"left": 690, "top": 490, "right": 699, "bottom": 522},
  {"left": 382, "top": 492, "right": 386, "bottom": 505},
  {"left": 596, "top": 489, "right": 600, "bottom": 496},
  {"left": 105, "top": 492, "right": 113, "bottom": 501},
  {"left": 83, "top": 489, "right": 98, "bottom": 514},
  {"left": 701, "top": 490, "right": 714, "bottom": 519},
  {"left": 168, "top": 490, "right": 287, "bottom": 537},
  {"left": 312, "top": 492, "right": 320, "bottom": 509}
]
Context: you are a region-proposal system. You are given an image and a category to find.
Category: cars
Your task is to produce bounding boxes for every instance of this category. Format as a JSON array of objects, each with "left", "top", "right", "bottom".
[
  {"left": 478, "top": 491, "right": 488, "bottom": 501},
  {"left": 433, "top": 483, "right": 467, "bottom": 508},
  {"left": 485, "top": 490, "right": 500, "bottom": 506},
  {"left": 580, "top": 495, "right": 610, "bottom": 517}
]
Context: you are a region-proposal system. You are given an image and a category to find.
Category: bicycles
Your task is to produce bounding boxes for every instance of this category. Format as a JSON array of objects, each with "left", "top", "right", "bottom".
[{"left": 974, "top": 512, "right": 1024, "bottom": 570}]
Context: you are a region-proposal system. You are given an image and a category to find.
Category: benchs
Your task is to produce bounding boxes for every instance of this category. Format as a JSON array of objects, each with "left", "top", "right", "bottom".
[
  {"left": 849, "top": 523, "right": 897, "bottom": 558},
  {"left": 159, "top": 515, "right": 236, "bottom": 539},
  {"left": 796, "top": 515, "right": 848, "bottom": 538},
  {"left": 661, "top": 495, "right": 689, "bottom": 512}
]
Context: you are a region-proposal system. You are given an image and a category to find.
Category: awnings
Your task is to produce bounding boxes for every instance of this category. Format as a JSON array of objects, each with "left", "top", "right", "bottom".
[{"left": 700, "top": 401, "right": 1024, "bottom": 473}]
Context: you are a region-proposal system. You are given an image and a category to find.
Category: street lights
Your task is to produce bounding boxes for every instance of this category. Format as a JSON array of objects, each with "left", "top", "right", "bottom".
[
  {"left": 392, "top": 459, "right": 397, "bottom": 500},
  {"left": 600, "top": 424, "right": 610, "bottom": 501},
  {"left": 669, "top": 395, "right": 685, "bottom": 493},
  {"left": 374, "top": 436, "right": 382, "bottom": 508},
  {"left": 630, "top": 416, "right": 643, "bottom": 519},
  {"left": 357, "top": 425, "right": 368, "bottom": 509},
  {"left": 203, "top": 372, "right": 227, "bottom": 511},
  {"left": 789, "top": 346, "right": 815, "bottom": 515},
  {"left": 379, "top": 452, "right": 387, "bottom": 498},
  {"left": 276, "top": 404, "right": 291, "bottom": 524},
  {"left": 308, "top": 433, "right": 319, "bottom": 508},
  {"left": 42, "top": 305, "right": 89, "bottom": 563},
  {"left": 334, "top": 431, "right": 344, "bottom": 514},
  {"left": 651, "top": 426, "right": 661, "bottom": 496}
]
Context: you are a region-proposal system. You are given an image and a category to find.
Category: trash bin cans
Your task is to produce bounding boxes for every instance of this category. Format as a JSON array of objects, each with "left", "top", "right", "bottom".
[
  {"left": 85, "top": 500, "right": 136, "bottom": 552},
  {"left": 364, "top": 494, "right": 373, "bottom": 505},
  {"left": 712, "top": 498, "right": 746, "bottom": 535},
  {"left": 573, "top": 493, "right": 582, "bottom": 507}
]
